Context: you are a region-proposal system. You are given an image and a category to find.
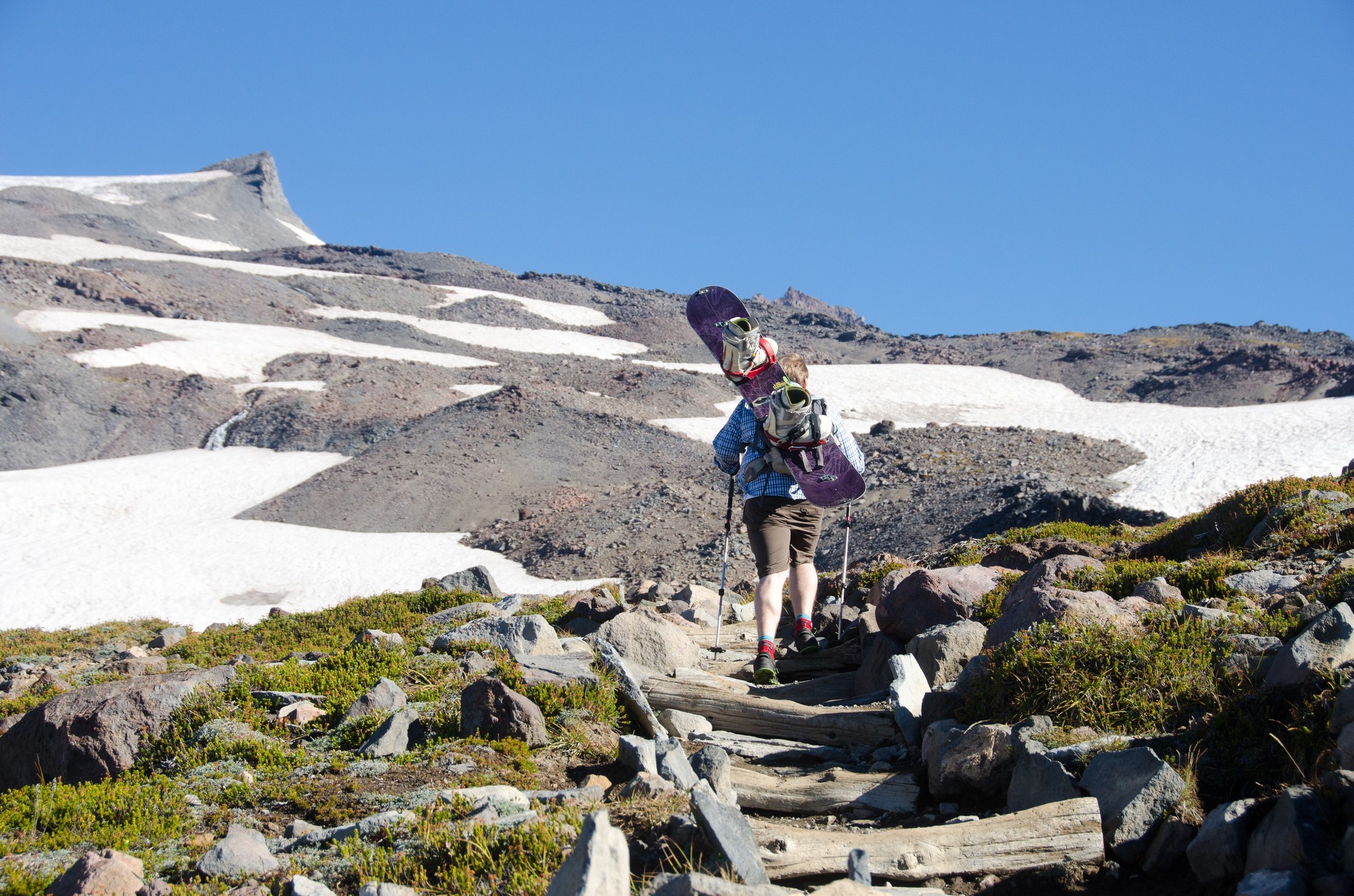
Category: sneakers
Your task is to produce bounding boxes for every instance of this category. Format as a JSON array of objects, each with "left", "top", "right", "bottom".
[
  {"left": 794, "top": 629, "right": 820, "bottom": 656},
  {"left": 752, "top": 652, "right": 781, "bottom": 684}
]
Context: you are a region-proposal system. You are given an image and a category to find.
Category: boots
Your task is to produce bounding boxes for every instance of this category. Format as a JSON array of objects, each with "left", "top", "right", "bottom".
[
  {"left": 763, "top": 386, "right": 832, "bottom": 444},
  {"left": 722, "top": 316, "right": 780, "bottom": 381}
]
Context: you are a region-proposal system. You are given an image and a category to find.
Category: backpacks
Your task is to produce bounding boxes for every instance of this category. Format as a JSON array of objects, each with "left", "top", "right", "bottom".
[{"left": 742, "top": 419, "right": 793, "bottom": 482}]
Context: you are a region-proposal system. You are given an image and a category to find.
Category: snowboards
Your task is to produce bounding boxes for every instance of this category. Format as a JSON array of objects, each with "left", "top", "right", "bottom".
[{"left": 685, "top": 285, "right": 867, "bottom": 508}]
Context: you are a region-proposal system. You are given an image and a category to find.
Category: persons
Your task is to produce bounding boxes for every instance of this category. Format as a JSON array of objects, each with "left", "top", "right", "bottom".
[{"left": 712, "top": 354, "right": 865, "bottom": 685}]
